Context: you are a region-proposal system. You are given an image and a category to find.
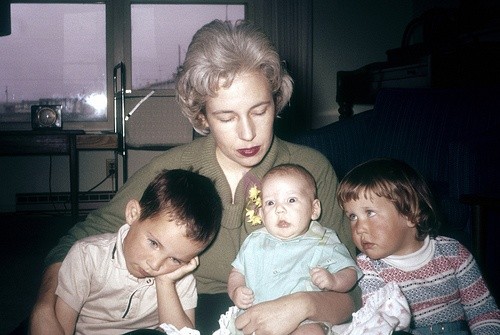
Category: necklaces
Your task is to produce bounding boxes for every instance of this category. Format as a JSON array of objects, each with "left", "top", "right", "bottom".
[{"left": 217, "top": 146, "right": 252, "bottom": 177}]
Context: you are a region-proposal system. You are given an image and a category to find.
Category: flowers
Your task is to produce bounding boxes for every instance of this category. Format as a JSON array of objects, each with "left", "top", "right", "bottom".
[{"left": 245, "top": 183, "right": 263, "bottom": 225}]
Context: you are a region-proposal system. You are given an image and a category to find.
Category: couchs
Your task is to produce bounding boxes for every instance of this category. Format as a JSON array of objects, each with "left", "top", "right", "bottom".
[{"left": 278, "top": 62, "right": 500, "bottom": 310}]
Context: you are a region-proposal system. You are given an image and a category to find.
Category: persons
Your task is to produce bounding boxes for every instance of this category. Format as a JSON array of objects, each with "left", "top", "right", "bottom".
[
  {"left": 335, "top": 158, "right": 500, "bottom": 335},
  {"left": 227, "top": 162, "right": 362, "bottom": 334},
  {"left": 53, "top": 166, "right": 224, "bottom": 334},
  {"left": 29, "top": 17, "right": 365, "bottom": 334}
]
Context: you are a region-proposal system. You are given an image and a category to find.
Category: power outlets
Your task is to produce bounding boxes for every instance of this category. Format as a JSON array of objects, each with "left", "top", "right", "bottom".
[{"left": 106, "top": 159, "right": 116, "bottom": 178}]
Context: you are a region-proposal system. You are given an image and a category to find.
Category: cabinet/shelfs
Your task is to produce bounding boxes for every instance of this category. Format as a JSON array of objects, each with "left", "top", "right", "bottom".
[{"left": 0, "top": 61, "right": 194, "bottom": 222}]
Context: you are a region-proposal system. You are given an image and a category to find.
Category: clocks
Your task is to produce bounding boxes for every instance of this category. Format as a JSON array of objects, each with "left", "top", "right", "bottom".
[{"left": 30, "top": 105, "right": 63, "bottom": 129}]
